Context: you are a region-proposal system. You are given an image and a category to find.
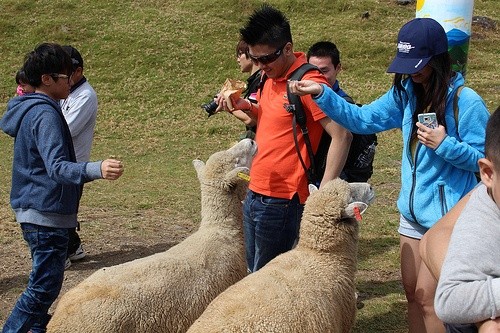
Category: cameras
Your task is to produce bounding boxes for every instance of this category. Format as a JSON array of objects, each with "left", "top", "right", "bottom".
[{"left": 201, "top": 95, "right": 218, "bottom": 119}]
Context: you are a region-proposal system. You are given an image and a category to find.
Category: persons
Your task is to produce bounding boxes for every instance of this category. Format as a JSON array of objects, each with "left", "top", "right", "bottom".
[
  {"left": 212, "top": 40, "right": 266, "bottom": 137},
  {"left": 418, "top": 108, "right": 500, "bottom": 333},
  {"left": 289, "top": 18, "right": 491, "bottom": 333},
  {"left": 17, "top": 70, "right": 36, "bottom": 95},
  {"left": 1, "top": 42, "right": 125, "bottom": 333},
  {"left": 17, "top": 42, "right": 97, "bottom": 270},
  {"left": 219, "top": 8, "right": 351, "bottom": 271},
  {"left": 307, "top": 40, "right": 354, "bottom": 184}
]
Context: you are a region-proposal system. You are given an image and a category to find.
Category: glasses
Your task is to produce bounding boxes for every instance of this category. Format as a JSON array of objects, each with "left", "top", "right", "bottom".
[
  {"left": 249, "top": 47, "right": 284, "bottom": 65},
  {"left": 51, "top": 73, "right": 73, "bottom": 84},
  {"left": 237, "top": 52, "right": 245, "bottom": 58}
]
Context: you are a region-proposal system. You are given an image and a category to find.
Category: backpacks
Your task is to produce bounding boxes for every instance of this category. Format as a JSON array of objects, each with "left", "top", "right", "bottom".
[{"left": 255, "top": 63, "right": 377, "bottom": 188}]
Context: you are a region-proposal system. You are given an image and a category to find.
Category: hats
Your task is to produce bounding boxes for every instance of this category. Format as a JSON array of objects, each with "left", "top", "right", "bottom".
[
  {"left": 61, "top": 45, "right": 83, "bottom": 67},
  {"left": 387, "top": 18, "right": 448, "bottom": 74}
]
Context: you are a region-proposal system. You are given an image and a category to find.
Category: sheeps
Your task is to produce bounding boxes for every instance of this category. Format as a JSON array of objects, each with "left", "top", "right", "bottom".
[
  {"left": 186, "top": 177, "right": 374, "bottom": 333},
  {"left": 46, "top": 138, "right": 258, "bottom": 333}
]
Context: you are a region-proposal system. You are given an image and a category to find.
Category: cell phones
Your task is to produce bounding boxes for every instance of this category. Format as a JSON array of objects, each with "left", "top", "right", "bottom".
[{"left": 417, "top": 112, "right": 439, "bottom": 130}]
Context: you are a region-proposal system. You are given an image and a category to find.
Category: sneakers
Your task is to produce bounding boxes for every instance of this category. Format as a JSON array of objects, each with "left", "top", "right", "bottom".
[
  {"left": 64, "top": 258, "right": 72, "bottom": 270},
  {"left": 69, "top": 245, "right": 84, "bottom": 260}
]
